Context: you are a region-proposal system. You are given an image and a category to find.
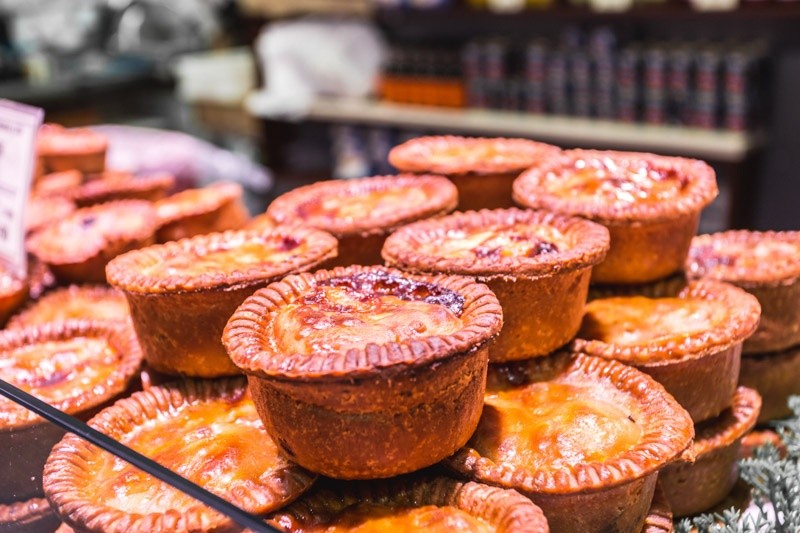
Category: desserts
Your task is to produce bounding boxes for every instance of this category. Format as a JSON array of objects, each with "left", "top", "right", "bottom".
[{"left": 2, "top": 54, "right": 799, "bottom": 527}]
